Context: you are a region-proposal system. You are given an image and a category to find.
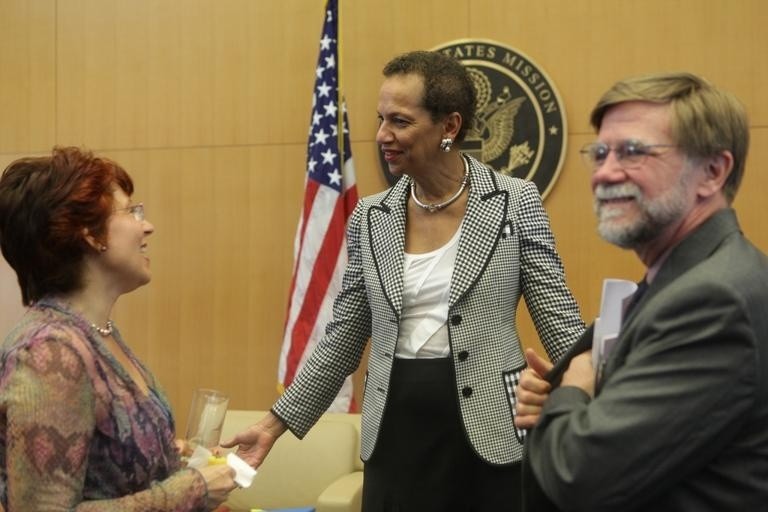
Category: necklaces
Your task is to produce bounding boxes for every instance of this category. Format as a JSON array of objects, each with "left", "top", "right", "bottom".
[
  {"left": 410, "top": 152, "right": 466, "bottom": 212},
  {"left": 90, "top": 319, "right": 113, "bottom": 336}
]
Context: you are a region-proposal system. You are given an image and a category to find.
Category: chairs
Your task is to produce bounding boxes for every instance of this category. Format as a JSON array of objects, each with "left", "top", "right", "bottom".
[{"left": 207, "top": 410, "right": 368, "bottom": 511}]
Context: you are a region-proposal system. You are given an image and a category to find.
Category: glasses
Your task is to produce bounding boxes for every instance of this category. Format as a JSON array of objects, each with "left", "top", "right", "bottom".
[
  {"left": 579, "top": 137, "right": 682, "bottom": 169},
  {"left": 113, "top": 203, "right": 145, "bottom": 221}
]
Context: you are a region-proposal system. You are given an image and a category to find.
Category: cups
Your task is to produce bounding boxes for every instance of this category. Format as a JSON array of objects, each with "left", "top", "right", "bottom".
[{"left": 181, "top": 388, "right": 232, "bottom": 469}]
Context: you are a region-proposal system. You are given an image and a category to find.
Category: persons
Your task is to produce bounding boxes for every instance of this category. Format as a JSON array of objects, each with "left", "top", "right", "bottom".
[
  {"left": 218, "top": 47, "right": 586, "bottom": 512},
  {"left": 512, "top": 71, "right": 768, "bottom": 512},
  {"left": 0, "top": 146, "right": 237, "bottom": 512}
]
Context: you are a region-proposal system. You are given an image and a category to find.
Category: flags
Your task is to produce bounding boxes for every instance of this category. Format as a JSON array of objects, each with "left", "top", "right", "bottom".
[{"left": 277, "top": 0, "right": 361, "bottom": 416}]
